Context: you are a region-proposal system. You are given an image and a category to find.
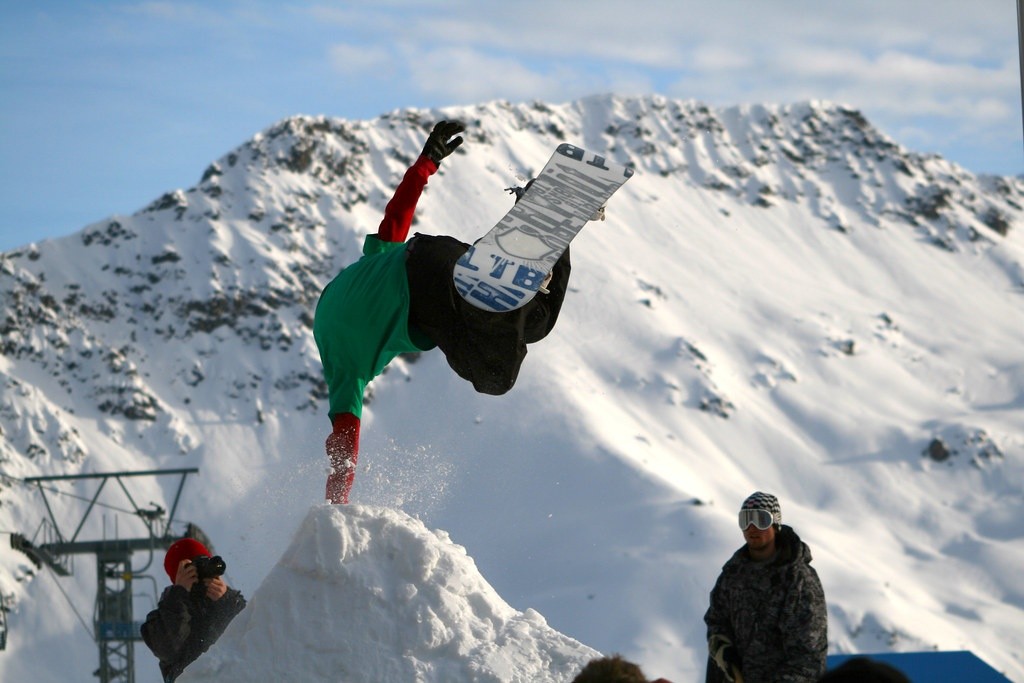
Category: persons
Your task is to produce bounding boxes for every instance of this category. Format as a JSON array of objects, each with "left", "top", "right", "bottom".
[
  {"left": 816, "top": 655, "right": 910, "bottom": 683},
  {"left": 703, "top": 491, "right": 829, "bottom": 683},
  {"left": 570, "top": 657, "right": 647, "bottom": 683},
  {"left": 312, "top": 120, "right": 571, "bottom": 501},
  {"left": 140, "top": 539, "right": 247, "bottom": 683}
]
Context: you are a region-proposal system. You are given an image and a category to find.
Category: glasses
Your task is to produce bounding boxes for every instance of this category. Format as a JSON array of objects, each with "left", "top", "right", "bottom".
[{"left": 738, "top": 509, "right": 773, "bottom": 531}]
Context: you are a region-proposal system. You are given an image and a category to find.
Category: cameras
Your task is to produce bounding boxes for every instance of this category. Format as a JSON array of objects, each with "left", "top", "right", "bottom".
[{"left": 184, "top": 555, "right": 226, "bottom": 591}]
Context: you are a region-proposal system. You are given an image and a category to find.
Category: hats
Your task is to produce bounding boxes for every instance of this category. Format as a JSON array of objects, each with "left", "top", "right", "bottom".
[
  {"left": 164, "top": 538, "right": 213, "bottom": 584},
  {"left": 741, "top": 492, "right": 782, "bottom": 526}
]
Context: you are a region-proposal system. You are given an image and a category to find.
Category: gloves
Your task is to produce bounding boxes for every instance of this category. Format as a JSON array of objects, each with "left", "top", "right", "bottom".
[
  {"left": 714, "top": 640, "right": 744, "bottom": 681},
  {"left": 421, "top": 120, "right": 465, "bottom": 162}
]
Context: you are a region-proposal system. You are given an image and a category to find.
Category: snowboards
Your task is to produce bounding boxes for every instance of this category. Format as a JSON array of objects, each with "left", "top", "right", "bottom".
[{"left": 452, "top": 141, "right": 637, "bottom": 312}]
